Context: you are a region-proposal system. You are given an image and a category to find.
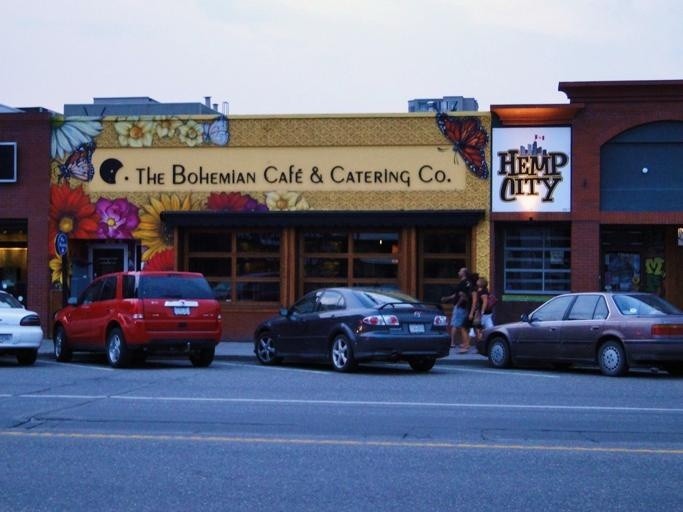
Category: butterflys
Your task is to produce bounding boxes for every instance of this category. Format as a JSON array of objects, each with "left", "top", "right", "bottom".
[
  {"left": 435, "top": 112, "right": 489, "bottom": 180},
  {"left": 202, "top": 115, "right": 230, "bottom": 145},
  {"left": 56, "top": 141, "right": 97, "bottom": 182}
]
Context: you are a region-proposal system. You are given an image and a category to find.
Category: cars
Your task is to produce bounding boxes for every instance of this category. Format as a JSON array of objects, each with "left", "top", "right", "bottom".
[
  {"left": 478, "top": 289, "right": 683, "bottom": 378},
  {"left": 0, "top": 289, "right": 45, "bottom": 364},
  {"left": 51, "top": 269, "right": 223, "bottom": 368},
  {"left": 254, "top": 288, "right": 452, "bottom": 374}
]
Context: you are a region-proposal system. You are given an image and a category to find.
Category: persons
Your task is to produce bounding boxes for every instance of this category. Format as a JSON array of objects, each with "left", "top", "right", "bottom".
[
  {"left": 441, "top": 268, "right": 478, "bottom": 352},
  {"left": 476, "top": 278, "right": 494, "bottom": 328}
]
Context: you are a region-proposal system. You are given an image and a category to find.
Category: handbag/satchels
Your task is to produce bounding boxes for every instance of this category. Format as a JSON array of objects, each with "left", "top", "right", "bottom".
[{"left": 482, "top": 294, "right": 497, "bottom": 310}]
